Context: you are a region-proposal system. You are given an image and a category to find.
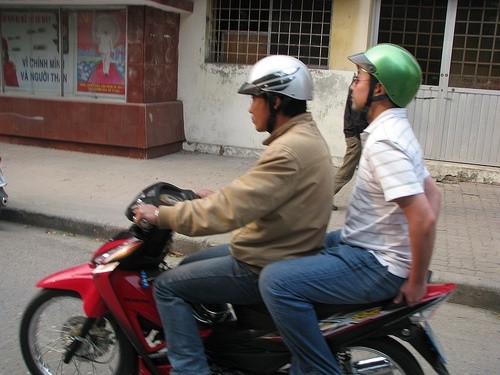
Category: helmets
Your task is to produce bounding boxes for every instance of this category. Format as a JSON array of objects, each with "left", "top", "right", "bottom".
[
  {"left": 348, "top": 43, "right": 423, "bottom": 108},
  {"left": 237, "top": 54, "right": 314, "bottom": 102}
]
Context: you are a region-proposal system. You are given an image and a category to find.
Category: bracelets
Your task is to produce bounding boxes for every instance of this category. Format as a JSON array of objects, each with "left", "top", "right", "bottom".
[{"left": 154, "top": 209, "right": 159, "bottom": 225}]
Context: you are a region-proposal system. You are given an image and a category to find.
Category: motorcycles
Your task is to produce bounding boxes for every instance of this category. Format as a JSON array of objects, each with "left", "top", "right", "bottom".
[{"left": 17, "top": 180, "right": 457, "bottom": 375}]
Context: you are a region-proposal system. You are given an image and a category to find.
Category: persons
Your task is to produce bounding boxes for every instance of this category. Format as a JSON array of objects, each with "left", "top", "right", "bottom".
[
  {"left": 322, "top": 85, "right": 371, "bottom": 212},
  {"left": 131, "top": 52, "right": 333, "bottom": 374},
  {"left": 258, "top": 44, "right": 442, "bottom": 374}
]
